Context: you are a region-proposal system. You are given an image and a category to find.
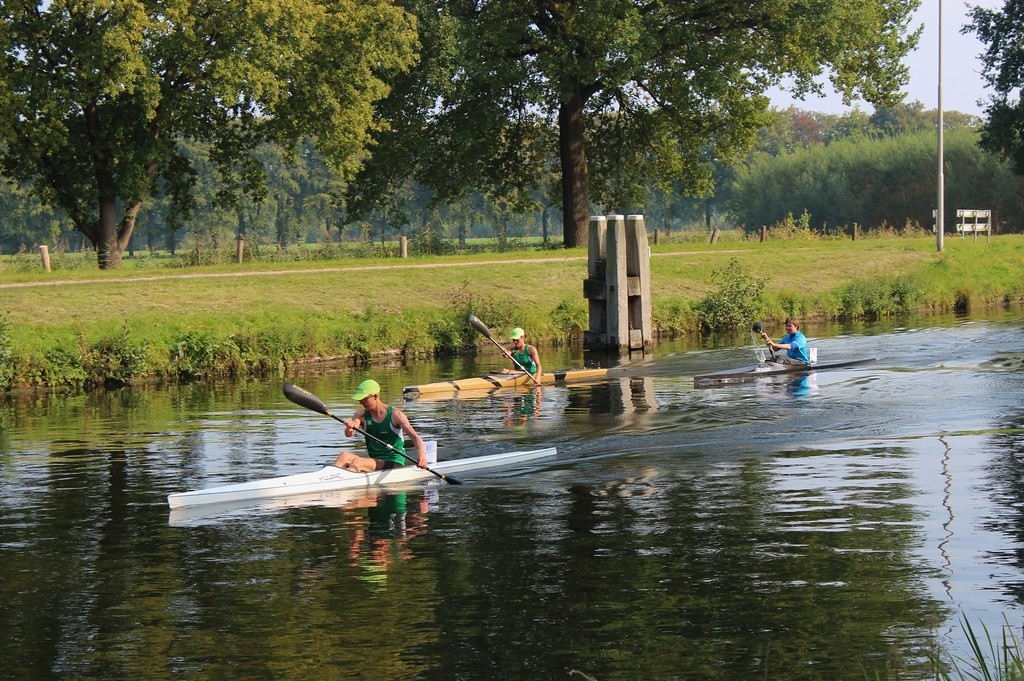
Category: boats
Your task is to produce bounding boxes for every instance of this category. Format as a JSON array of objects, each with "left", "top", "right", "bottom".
[
  {"left": 693, "top": 358, "right": 877, "bottom": 388},
  {"left": 168, "top": 446, "right": 558, "bottom": 510},
  {"left": 401, "top": 369, "right": 609, "bottom": 395}
]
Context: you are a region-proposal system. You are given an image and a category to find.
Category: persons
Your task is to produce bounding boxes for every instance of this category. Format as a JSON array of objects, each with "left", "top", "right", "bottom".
[
  {"left": 761, "top": 316, "right": 809, "bottom": 365},
  {"left": 501, "top": 327, "right": 542, "bottom": 386},
  {"left": 335, "top": 379, "right": 427, "bottom": 473}
]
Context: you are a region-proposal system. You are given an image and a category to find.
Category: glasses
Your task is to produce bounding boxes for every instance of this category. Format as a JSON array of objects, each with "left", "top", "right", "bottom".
[{"left": 358, "top": 395, "right": 372, "bottom": 401}]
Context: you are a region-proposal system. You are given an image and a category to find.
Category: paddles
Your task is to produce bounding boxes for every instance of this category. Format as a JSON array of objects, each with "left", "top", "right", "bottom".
[
  {"left": 283, "top": 381, "right": 463, "bottom": 485},
  {"left": 752, "top": 322, "right": 776, "bottom": 362},
  {"left": 469, "top": 315, "right": 542, "bottom": 385}
]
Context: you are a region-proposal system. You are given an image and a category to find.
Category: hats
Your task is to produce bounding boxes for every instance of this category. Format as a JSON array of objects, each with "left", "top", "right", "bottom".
[
  {"left": 351, "top": 379, "right": 380, "bottom": 400},
  {"left": 509, "top": 328, "right": 524, "bottom": 339}
]
